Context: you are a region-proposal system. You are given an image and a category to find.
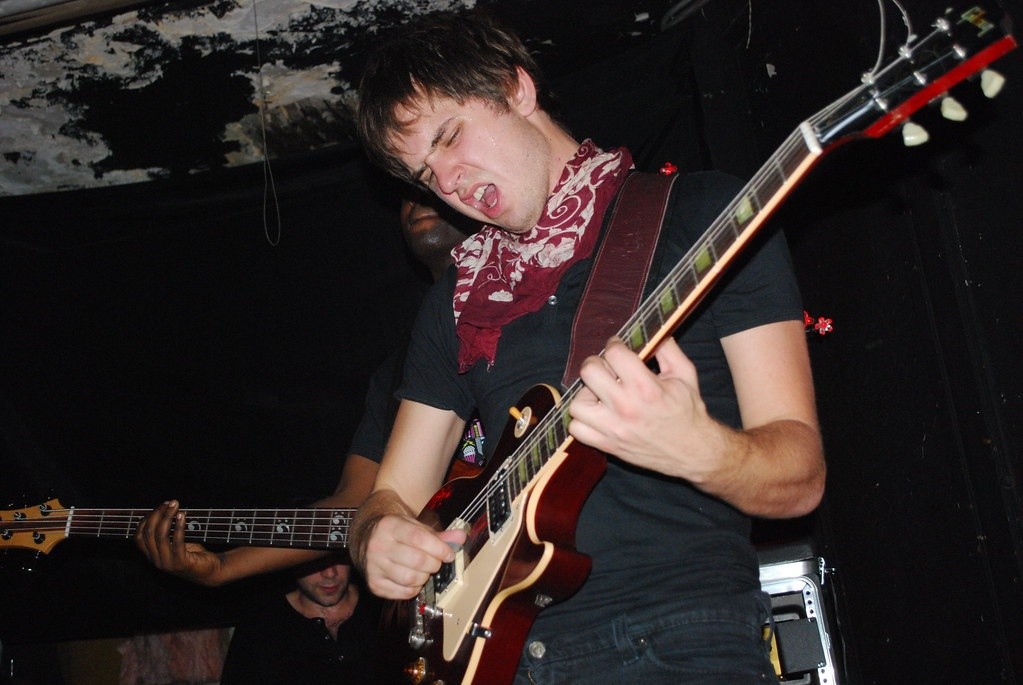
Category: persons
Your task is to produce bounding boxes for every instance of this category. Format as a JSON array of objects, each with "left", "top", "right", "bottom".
[
  {"left": 222, "top": 499, "right": 427, "bottom": 685},
  {"left": 346, "top": 7, "right": 827, "bottom": 685},
  {"left": 133, "top": 192, "right": 486, "bottom": 685}
]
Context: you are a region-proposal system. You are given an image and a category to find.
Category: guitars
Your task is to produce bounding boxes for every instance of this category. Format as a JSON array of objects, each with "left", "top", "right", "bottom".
[
  {"left": 1, "top": 483, "right": 359, "bottom": 573},
  {"left": 369, "top": 2, "right": 1017, "bottom": 685}
]
